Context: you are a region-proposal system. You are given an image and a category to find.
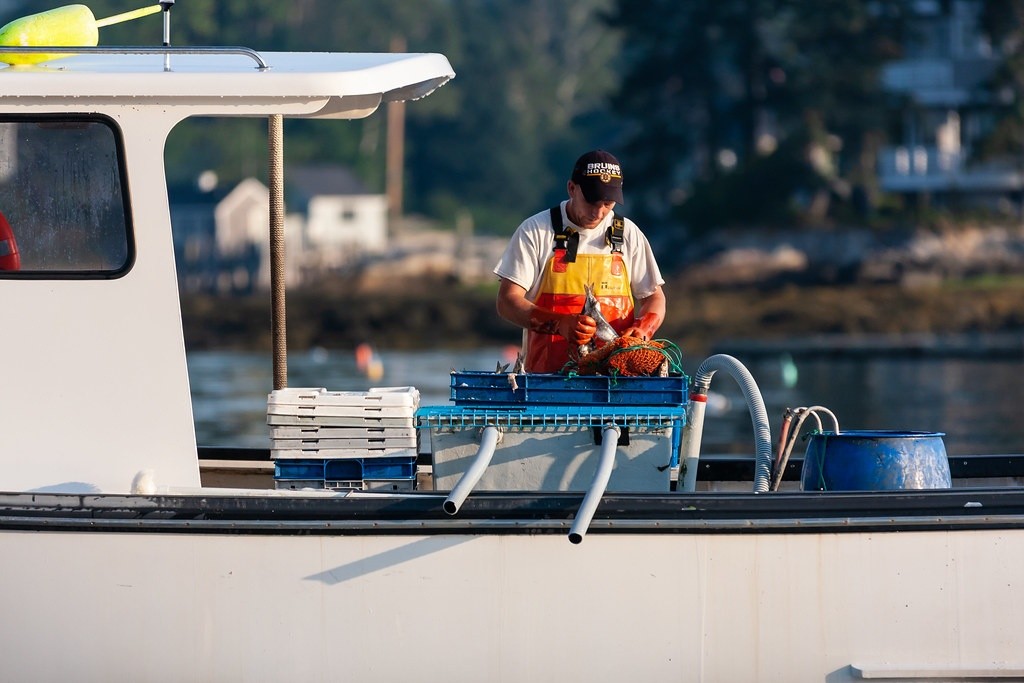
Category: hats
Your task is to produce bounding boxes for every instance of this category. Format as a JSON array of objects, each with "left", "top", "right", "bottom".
[{"left": 571, "top": 149, "right": 624, "bottom": 206}]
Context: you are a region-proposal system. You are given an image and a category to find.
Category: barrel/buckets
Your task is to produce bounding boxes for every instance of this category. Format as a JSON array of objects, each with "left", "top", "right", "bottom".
[{"left": 799, "top": 430, "right": 953, "bottom": 490}]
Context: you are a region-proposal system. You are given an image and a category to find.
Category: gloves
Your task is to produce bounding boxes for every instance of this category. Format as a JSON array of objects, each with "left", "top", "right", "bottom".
[
  {"left": 623, "top": 312, "right": 661, "bottom": 342},
  {"left": 529, "top": 308, "right": 597, "bottom": 345}
]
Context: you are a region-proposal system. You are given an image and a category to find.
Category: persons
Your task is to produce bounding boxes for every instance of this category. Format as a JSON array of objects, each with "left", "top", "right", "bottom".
[{"left": 493, "top": 149, "right": 666, "bottom": 373}]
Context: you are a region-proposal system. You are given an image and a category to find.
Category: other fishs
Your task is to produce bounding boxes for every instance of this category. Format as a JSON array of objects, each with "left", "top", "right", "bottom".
[
  {"left": 657, "top": 357, "right": 670, "bottom": 378},
  {"left": 577, "top": 283, "right": 618, "bottom": 357},
  {"left": 495, "top": 351, "right": 527, "bottom": 374}
]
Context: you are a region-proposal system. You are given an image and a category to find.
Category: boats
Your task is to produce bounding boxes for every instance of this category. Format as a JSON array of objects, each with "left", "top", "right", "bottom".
[{"left": 0, "top": 0, "right": 1024, "bottom": 683}]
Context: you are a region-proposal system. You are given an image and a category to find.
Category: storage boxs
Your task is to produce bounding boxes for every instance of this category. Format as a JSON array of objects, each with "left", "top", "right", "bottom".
[
  {"left": 266, "top": 384, "right": 421, "bottom": 498},
  {"left": 425, "top": 412, "right": 673, "bottom": 493},
  {"left": 449, "top": 370, "right": 692, "bottom": 406}
]
